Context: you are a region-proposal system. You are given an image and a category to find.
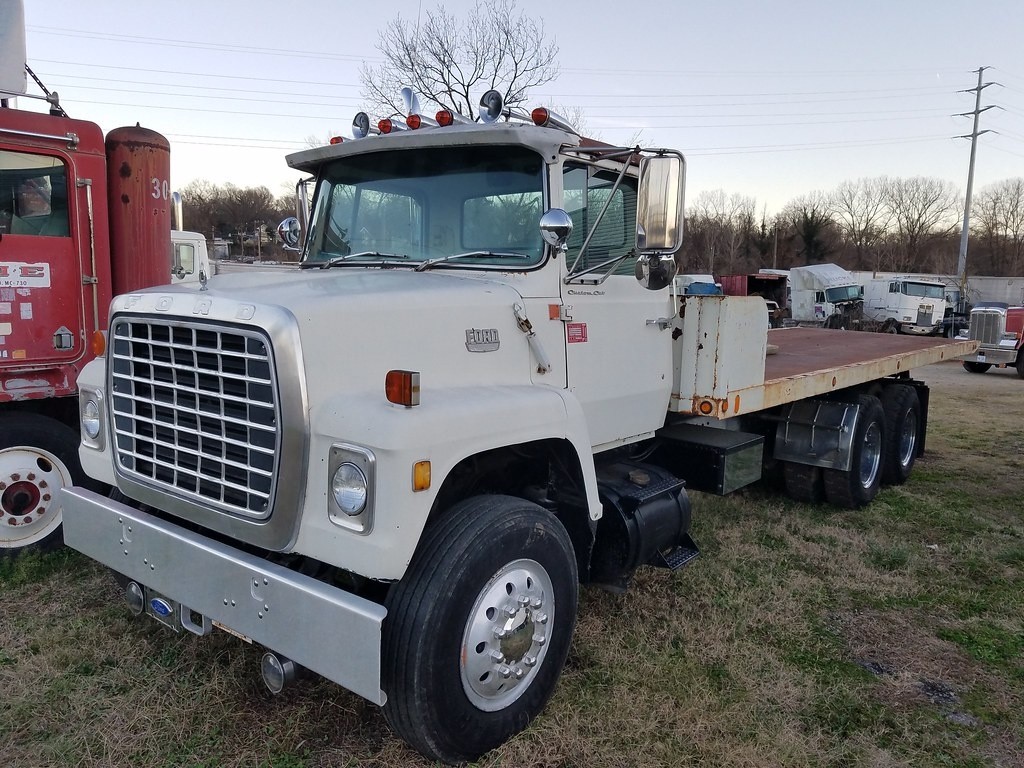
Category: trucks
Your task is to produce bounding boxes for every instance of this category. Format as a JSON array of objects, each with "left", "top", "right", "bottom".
[
  {"left": 0, "top": 0, "right": 300, "bottom": 559},
  {"left": 670, "top": 265, "right": 967, "bottom": 335},
  {"left": 59, "top": 87, "right": 981, "bottom": 764},
  {"left": 953, "top": 306, "right": 1024, "bottom": 379}
]
